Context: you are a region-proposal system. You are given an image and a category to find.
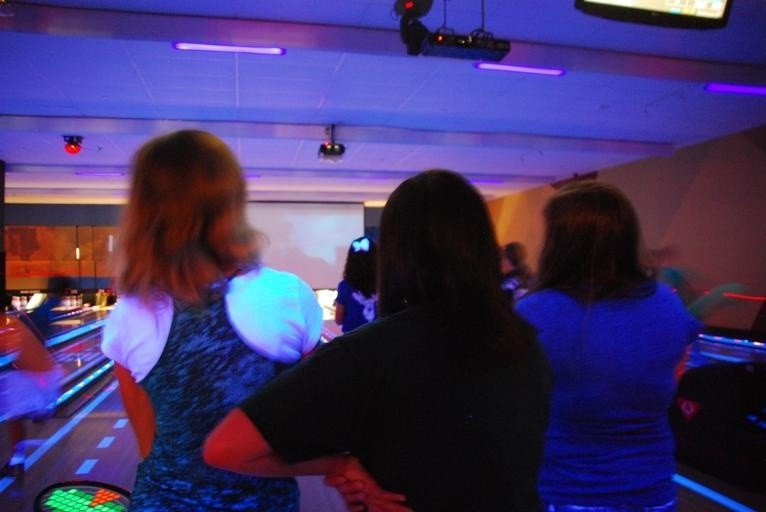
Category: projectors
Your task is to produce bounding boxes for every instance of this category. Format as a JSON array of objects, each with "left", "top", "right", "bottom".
[{"left": 318, "top": 143, "right": 344, "bottom": 160}]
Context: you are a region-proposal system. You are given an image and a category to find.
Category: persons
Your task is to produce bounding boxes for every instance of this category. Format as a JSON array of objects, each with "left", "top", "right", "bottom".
[
  {"left": 204, "top": 167, "right": 550, "bottom": 512},
  {"left": 97, "top": 128, "right": 325, "bottom": 512},
  {"left": 500, "top": 242, "right": 541, "bottom": 300},
  {"left": 334, "top": 236, "right": 380, "bottom": 333},
  {"left": 0, "top": 272, "right": 76, "bottom": 482},
  {"left": 511, "top": 180, "right": 707, "bottom": 512}
]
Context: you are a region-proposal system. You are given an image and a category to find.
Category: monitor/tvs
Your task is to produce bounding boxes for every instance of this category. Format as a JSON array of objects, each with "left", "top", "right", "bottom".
[{"left": 575, "top": 0, "right": 735, "bottom": 30}]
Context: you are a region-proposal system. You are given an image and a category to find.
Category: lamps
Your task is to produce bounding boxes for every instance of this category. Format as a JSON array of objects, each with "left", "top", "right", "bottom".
[
  {"left": 60, "top": 134, "right": 85, "bottom": 155},
  {"left": 171, "top": 38, "right": 287, "bottom": 58}
]
